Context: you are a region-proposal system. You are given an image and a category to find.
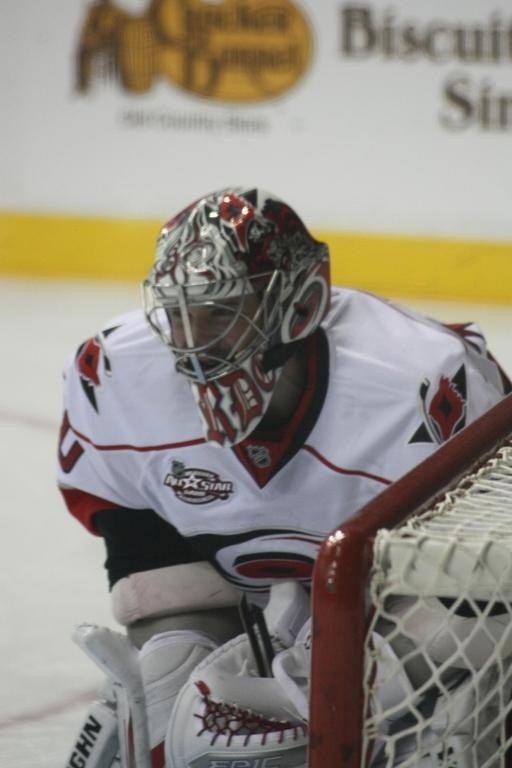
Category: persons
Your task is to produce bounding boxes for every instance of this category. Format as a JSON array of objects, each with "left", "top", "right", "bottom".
[{"left": 55, "top": 184, "right": 511, "bottom": 768}]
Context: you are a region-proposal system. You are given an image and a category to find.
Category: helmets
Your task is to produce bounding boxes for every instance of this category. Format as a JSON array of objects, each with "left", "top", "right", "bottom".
[{"left": 142, "top": 187, "right": 331, "bottom": 448}]
